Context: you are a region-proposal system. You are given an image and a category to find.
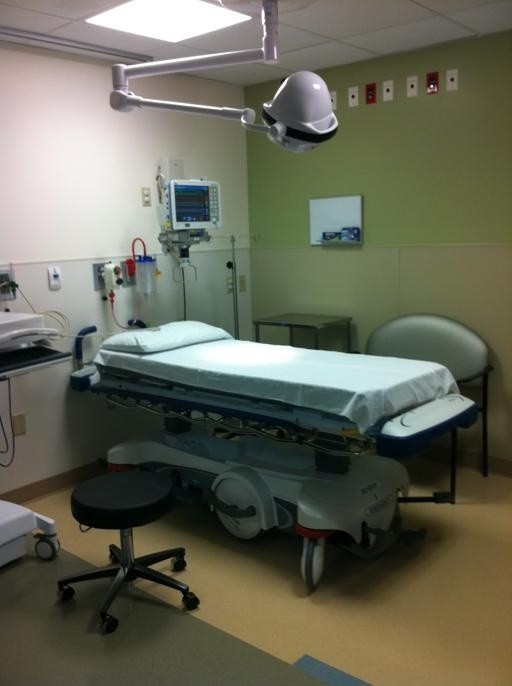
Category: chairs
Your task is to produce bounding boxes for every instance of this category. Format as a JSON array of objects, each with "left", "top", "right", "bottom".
[{"left": 365, "top": 311, "right": 496, "bottom": 477}]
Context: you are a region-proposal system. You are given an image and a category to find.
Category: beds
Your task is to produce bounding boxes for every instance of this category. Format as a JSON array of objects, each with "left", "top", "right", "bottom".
[{"left": 76, "top": 318, "right": 464, "bottom": 592}]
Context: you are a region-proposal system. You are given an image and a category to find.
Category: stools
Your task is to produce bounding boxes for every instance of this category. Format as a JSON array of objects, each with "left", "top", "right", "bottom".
[{"left": 60, "top": 473, "right": 199, "bottom": 634}]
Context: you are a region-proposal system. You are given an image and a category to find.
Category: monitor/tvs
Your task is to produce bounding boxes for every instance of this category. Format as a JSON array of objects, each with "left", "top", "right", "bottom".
[{"left": 169, "top": 179, "right": 222, "bottom": 230}]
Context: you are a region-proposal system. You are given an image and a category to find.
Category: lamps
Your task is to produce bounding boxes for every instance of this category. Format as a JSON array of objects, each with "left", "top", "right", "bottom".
[{"left": 110, "top": 4, "right": 341, "bottom": 157}]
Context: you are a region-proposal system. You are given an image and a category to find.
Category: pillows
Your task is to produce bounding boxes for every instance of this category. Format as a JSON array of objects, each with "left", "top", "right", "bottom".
[{"left": 99, "top": 319, "right": 235, "bottom": 356}]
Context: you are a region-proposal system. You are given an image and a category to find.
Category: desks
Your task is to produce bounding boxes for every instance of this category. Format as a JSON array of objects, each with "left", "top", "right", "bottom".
[{"left": 254, "top": 311, "right": 353, "bottom": 350}]
